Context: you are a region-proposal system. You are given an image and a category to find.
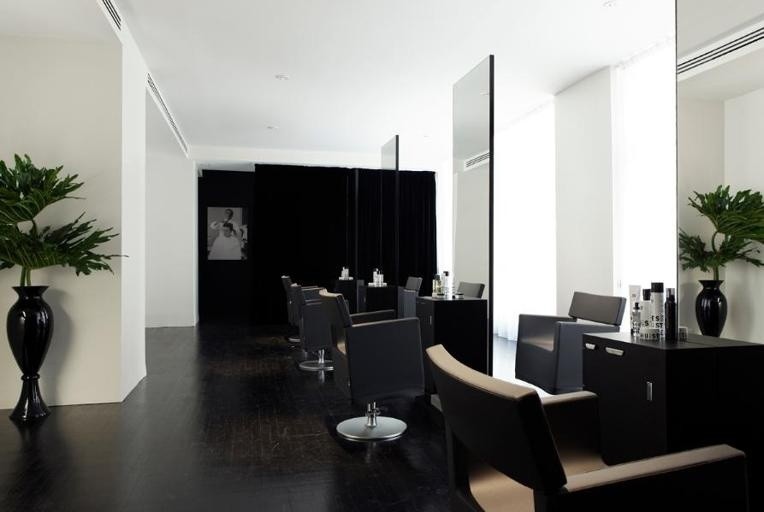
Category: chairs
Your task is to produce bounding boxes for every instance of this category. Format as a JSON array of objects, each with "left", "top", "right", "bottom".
[
  {"left": 281, "top": 276, "right": 323, "bottom": 342},
  {"left": 515, "top": 291, "right": 626, "bottom": 395},
  {"left": 426, "top": 344, "right": 745, "bottom": 512},
  {"left": 291, "top": 283, "right": 349, "bottom": 371},
  {"left": 398, "top": 277, "right": 422, "bottom": 318},
  {"left": 319, "top": 289, "right": 425, "bottom": 444},
  {"left": 457, "top": 282, "right": 485, "bottom": 298}
]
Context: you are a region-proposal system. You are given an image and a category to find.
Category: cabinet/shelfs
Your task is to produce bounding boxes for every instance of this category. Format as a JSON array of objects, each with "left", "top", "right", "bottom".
[
  {"left": 416, "top": 297, "right": 487, "bottom": 394},
  {"left": 582, "top": 334, "right": 764, "bottom": 466},
  {"left": 358, "top": 285, "right": 398, "bottom": 312}
]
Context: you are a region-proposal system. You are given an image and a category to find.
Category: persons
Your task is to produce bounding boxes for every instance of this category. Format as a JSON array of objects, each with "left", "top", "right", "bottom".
[
  {"left": 209, "top": 209, "right": 241, "bottom": 241},
  {"left": 207, "top": 222, "right": 241, "bottom": 260}
]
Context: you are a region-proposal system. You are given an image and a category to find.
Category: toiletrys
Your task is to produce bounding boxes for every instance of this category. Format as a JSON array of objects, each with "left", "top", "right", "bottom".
[
  {"left": 632, "top": 303, "right": 642, "bottom": 333},
  {"left": 651, "top": 281, "right": 664, "bottom": 335},
  {"left": 340, "top": 265, "right": 349, "bottom": 280},
  {"left": 639, "top": 326, "right": 660, "bottom": 342},
  {"left": 665, "top": 288, "right": 676, "bottom": 342},
  {"left": 433, "top": 271, "right": 453, "bottom": 299},
  {"left": 373, "top": 268, "right": 383, "bottom": 284},
  {"left": 679, "top": 326, "right": 688, "bottom": 342},
  {"left": 641, "top": 289, "right": 652, "bottom": 325}
]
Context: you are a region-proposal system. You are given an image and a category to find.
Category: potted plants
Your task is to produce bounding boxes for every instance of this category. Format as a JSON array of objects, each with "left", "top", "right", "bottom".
[
  {"left": 0, "top": 152, "right": 129, "bottom": 421},
  {"left": 678, "top": 184, "right": 764, "bottom": 337}
]
